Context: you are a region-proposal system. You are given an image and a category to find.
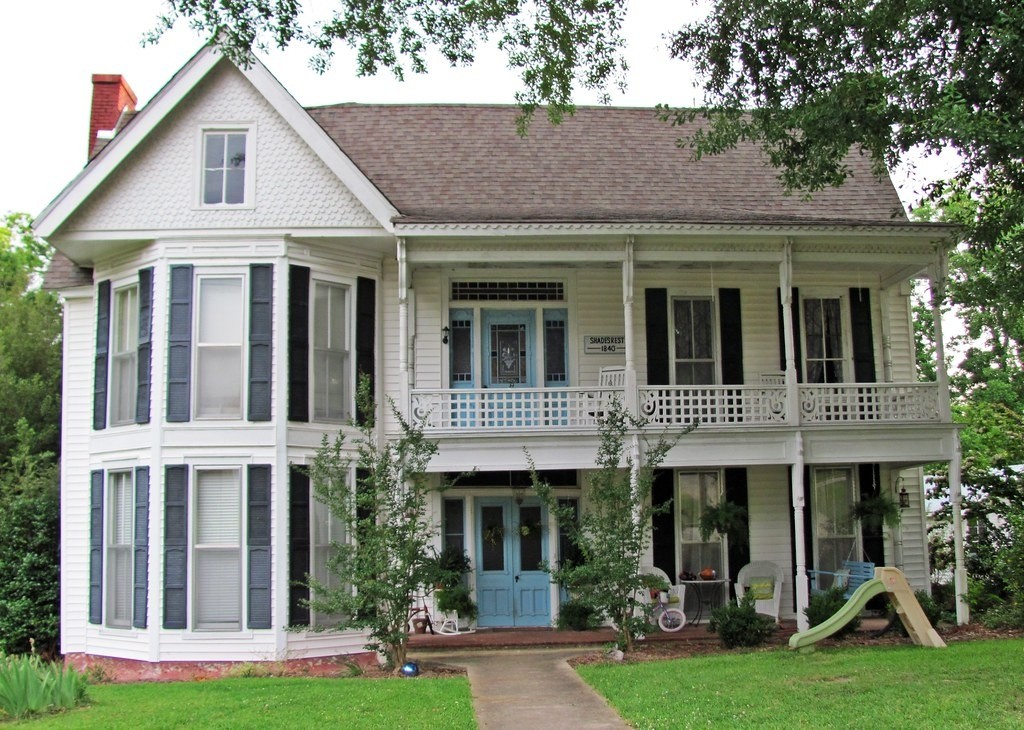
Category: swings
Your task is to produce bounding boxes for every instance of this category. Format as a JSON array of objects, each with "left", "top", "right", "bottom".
[{"left": 806, "top": 536, "right": 876, "bottom": 601}]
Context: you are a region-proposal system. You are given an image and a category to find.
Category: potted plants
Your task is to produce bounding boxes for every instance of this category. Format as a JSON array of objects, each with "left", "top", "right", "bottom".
[
  {"left": 849, "top": 489, "right": 905, "bottom": 528},
  {"left": 697, "top": 498, "right": 749, "bottom": 545},
  {"left": 433, "top": 543, "right": 473, "bottom": 588}
]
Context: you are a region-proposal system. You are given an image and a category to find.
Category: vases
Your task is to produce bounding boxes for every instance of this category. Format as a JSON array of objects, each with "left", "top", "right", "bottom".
[{"left": 411, "top": 618, "right": 428, "bottom": 634}]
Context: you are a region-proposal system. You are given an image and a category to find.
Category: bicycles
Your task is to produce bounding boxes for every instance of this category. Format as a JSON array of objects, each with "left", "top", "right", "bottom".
[
  {"left": 633, "top": 586, "right": 687, "bottom": 640},
  {"left": 396, "top": 584, "right": 445, "bottom": 635}
]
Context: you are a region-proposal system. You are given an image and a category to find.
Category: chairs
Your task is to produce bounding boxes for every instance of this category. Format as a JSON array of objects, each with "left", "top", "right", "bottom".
[
  {"left": 432, "top": 608, "right": 477, "bottom": 635},
  {"left": 760, "top": 369, "right": 786, "bottom": 402},
  {"left": 589, "top": 362, "right": 626, "bottom": 421},
  {"left": 628, "top": 565, "right": 685, "bottom": 624},
  {"left": 734, "top": 560, "right": 783, "bottom": 626}
]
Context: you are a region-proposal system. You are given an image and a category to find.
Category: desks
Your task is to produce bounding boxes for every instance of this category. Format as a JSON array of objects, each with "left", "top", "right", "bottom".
[{"left": 682, "top": 578, "right": 730, "bottom": 627}]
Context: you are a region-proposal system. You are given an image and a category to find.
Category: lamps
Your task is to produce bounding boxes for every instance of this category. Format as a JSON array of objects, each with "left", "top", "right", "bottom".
[
  {"left": 441, "top": 325, "right": 450, "bottom": 346},
  {"left": 895, "top": 476, "right": 910, "bottom": 508}
]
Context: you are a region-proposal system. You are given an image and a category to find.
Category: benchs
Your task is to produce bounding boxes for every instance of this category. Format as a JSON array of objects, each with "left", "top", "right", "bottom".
[{"left": 807, "top": 560, "right": 875, "bottom": 600}]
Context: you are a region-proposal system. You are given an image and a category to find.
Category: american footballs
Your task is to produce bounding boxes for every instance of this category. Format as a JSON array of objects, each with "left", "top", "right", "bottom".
[{"left": 700, "top": 567, "right": 718, "bottom": 580}]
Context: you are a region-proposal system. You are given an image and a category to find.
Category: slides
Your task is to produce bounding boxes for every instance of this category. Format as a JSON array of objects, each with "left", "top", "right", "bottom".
[{"left": 788, "top": 577, "right": 887, "bottom": 649}]
